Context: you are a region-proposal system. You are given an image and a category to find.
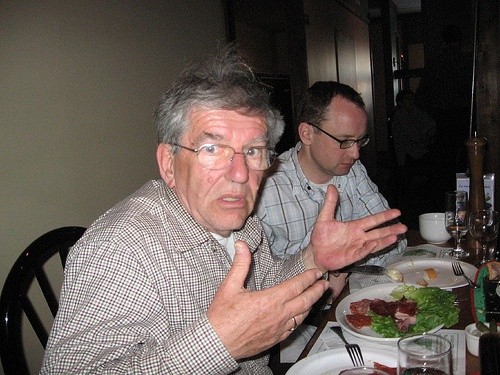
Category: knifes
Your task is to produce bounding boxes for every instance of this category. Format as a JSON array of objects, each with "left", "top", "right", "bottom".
[{"left": 334, "top": 265, "right": 388, "bottom": 277}]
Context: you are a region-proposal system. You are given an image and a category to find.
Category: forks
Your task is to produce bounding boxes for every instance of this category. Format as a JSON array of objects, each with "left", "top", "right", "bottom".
[
  {"left": 329, "top": 326, "right": 364, "bottom": 367},
  {"left": 451, "top": 262, "right": 480, "bottom": 289}
]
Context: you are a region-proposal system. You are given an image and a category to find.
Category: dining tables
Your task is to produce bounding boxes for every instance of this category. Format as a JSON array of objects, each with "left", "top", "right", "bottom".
[{"left": 295, "top": 227, "right": 500, "bottom": 375}]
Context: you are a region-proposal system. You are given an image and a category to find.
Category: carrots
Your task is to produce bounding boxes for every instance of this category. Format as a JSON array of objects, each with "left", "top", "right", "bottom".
[{"left": 424, "top": 268, "right": 436, "bottom": 279}]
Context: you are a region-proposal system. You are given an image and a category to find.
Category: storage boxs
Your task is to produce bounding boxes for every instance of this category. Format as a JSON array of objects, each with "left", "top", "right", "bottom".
[{"left": 455, "top": 171, "right": 496, "bottom": 222}]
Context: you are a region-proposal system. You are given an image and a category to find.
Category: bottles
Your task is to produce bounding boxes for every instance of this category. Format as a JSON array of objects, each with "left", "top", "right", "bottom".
[{"left": 465, "top": 131, "right": 486, "bottom": 259}]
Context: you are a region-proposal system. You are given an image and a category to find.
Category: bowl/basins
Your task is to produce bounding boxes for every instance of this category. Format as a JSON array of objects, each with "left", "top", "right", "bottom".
[
  {"left": 465, "top": 322, "right": 500, "bottom": 357},
  {"left": 419, "top": 213, "right": 451, "bottom": 244}
]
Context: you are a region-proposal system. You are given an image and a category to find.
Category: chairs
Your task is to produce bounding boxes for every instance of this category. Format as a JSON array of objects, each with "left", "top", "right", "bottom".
[{"left": 0, "top": 225, "right": 87, "bottom": 375}]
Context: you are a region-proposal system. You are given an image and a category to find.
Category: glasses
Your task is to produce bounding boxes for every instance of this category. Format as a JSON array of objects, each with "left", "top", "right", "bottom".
[
  {"left": 302, "top": 119, "right": 371, "bottom": 149},
  {"left": 163, "top": 140, "right": 278, "bottom": 171}
]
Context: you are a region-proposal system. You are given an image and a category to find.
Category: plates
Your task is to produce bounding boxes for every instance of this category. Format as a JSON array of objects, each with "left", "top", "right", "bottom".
[
  {"left": 384, "top": 259, "right": 478, "bottom": 289},
  {"left": 335, "top": 282, "right": 444, "bottom": 342},
  {"left": 285, "top": 346, "right": 405, "bottom": 375}
]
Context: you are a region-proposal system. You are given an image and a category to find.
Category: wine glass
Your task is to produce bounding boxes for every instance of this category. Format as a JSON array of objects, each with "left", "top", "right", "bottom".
[
  {"left": 469, "top": 209, "right": 500, "bottom": 266},
  {"left": 445, "top": 191, "right": 470, "bottom": 258}
]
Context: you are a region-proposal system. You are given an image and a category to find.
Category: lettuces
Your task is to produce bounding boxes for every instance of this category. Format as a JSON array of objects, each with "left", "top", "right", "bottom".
[{"left": 367, "top": 285, "right": 461, "bottom": 338}]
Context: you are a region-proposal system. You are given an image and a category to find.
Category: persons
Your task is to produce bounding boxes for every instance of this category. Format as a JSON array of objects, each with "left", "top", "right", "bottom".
[
  {"left": 392, "top": 87, "right": 437, "bottom": 230},
  {"left": 255, "top": 81, "right": 407, "bottom": 363},
  {"left": 38, "top": 36, "right": 407, "bottom": 375}
]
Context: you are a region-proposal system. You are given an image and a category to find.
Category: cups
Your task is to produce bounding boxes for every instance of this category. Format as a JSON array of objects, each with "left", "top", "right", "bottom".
[
  {"left": 397, "top": 334, "right": 453, "bottom": 375},
  {"left": 338, "top": 367, "right": 389, "bottom": 375},
  {"left": 479, "top": 333, "right": 500, "bottom": 375},
  {"left": 483, "top": 275, "right": 500, "bottom": 322}
]
